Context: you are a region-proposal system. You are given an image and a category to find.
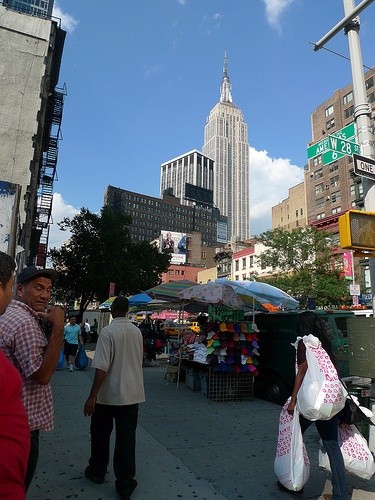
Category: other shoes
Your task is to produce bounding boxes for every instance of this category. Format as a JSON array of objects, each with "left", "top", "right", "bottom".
[
  {"left": 69, "top": 365, "right": 74, "bottom": 372},
  {"left": 67, "top": 363, "right": 69, "bottom": 368},
  {"left": 84, "top": 466, "right": 104, "bottom": 484}
]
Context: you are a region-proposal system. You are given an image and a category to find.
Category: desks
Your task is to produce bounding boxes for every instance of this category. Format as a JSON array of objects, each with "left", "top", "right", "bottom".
[{"left": 169, "top": 354, "right": 210, "bottom": 384}]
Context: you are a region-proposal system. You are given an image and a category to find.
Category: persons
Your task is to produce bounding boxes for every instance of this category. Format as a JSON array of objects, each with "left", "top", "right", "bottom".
[
  {"left": 0, "top": 250, "right": 31, "bottom": 500},
  {"left": 277, "top": 312, "right": 349, "bottom": 500},
  {"left": 83, "top": 295, "right": 145, "bottom": 500},
  {"left": 39, "top": 304, "right": 98, "bottom": 372},
  {"left": 162, "top": 232, "right": 187, "bottom": 254},
  {"left": 0, "top": 266, "right": 64, "bottom": 494},
  {"left": 138, "top": 313, "right": 156, "bottom": 367}
]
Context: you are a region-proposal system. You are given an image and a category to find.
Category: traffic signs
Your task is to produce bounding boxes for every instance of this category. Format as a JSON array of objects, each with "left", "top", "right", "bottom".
[{"left": 352, "top": 153, "right": 375, "bottom": 182}]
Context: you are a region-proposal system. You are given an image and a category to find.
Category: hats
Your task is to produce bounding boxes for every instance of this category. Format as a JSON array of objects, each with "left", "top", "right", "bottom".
[
  {"left": 201, "top": 322, "right": 260, "bottom": 376},
  {"left": 16, "top": 266, "right": 58, "bottom": 284}
]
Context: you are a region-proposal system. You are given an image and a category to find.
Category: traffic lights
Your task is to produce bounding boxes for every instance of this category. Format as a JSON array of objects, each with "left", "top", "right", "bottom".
[{"left": 338, "top": 208, "right": 375, "bottom": 259}]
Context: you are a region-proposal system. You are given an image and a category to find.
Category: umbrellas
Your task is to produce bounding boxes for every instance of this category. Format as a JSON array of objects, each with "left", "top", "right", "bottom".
[{"left": 97, "top": 276, "right": 300, "bottom": 396}]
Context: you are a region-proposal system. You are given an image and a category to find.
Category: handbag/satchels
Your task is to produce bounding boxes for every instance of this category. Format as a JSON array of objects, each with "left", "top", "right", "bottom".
[
  {"left": 75, "top": 345, "right": 88, "bottom": 368},
  {"left": 340, "top": 398, "right": 363, "bottom": 424}
]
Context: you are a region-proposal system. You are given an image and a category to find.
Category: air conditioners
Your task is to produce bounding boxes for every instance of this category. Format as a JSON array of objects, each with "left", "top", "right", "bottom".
[{"left": 325, "top": 196, "right": 329, "bottom": 199}]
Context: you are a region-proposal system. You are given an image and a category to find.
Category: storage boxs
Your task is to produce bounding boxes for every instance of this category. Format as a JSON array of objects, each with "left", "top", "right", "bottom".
[{"left": 185, "top": 368, "right": 227, "bottom": 396}]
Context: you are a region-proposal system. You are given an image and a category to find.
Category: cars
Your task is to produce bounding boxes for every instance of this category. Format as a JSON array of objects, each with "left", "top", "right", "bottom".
[{"left": 151, "top": 319, "right": 201, "bottom": 337}]
